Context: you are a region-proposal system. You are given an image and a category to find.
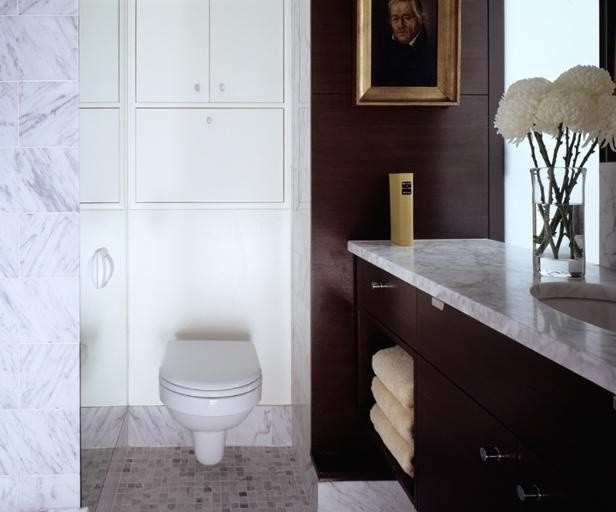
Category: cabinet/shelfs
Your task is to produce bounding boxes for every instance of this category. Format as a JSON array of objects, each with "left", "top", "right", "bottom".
[
  {"left": 355, "top": 253, "right": 616, "bottom": 509},
  {"left": 77, "top": 1, "right": 119, "bottom": 405},
  {"left": 135, "top": 1, "right": 283, "bottom": 203}
]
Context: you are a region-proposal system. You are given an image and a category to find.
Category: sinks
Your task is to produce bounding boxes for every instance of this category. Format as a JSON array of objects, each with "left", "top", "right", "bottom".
[{"left": 530, "top": 281, "right": 616, "bottom": 334}]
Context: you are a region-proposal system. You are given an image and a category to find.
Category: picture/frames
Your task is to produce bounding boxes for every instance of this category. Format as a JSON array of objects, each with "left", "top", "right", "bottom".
[{"left": 355, "top": 0, "right": 461, "bottom": 107}]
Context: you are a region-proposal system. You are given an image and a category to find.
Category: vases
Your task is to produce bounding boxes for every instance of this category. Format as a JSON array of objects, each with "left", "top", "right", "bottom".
[{"left": 492, "top": 64, "right": 616, "bottom": 279}]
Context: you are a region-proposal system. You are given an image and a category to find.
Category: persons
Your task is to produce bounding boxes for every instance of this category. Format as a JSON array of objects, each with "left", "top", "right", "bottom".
[{"left": 377, "top": 1, "right": 437, "bottom": 86}]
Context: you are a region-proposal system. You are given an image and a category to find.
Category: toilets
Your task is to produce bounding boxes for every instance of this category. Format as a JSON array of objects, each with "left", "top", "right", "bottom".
[{"left": 158, "top": 339, "right": 263, "bottom": 465}]
